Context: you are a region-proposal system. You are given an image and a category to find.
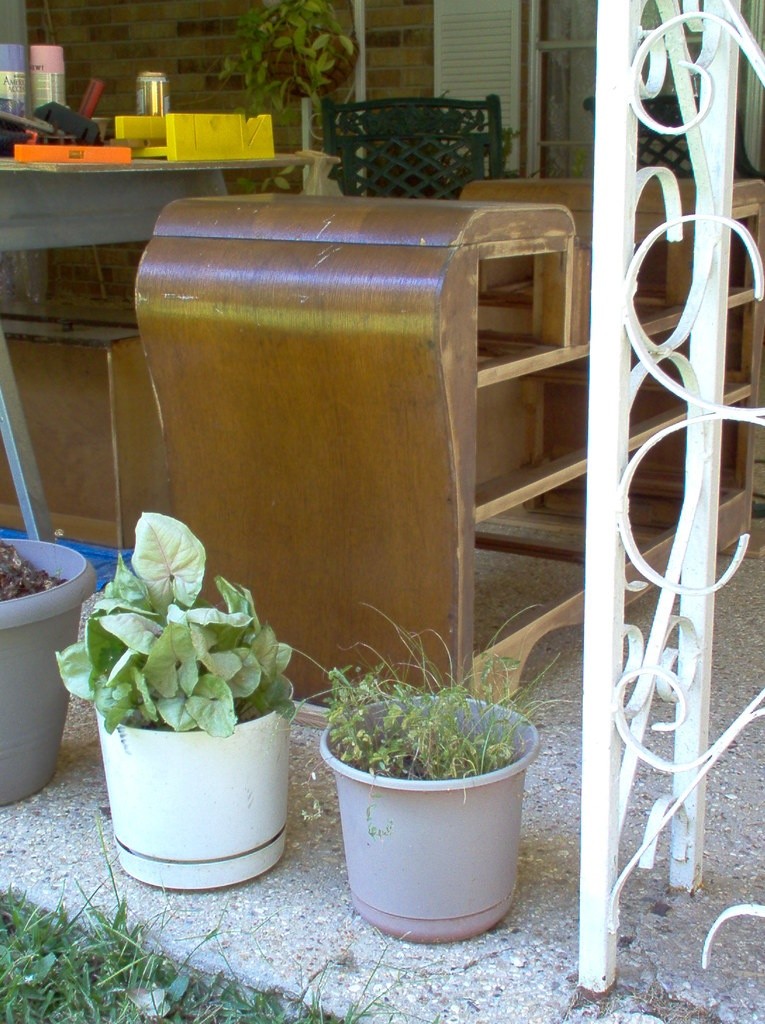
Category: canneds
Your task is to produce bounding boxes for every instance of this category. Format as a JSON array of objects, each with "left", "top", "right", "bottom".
[{"left": 136, "top": 71, "right": 170, "bottom": 116}]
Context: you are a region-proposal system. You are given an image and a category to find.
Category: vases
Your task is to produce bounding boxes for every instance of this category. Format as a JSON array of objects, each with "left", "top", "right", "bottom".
[{"left": 0, "top": 537, "right": 94, "bottom": 809}]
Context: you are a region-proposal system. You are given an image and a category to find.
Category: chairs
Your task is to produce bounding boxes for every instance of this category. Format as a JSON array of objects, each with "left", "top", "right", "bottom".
[
  {"left": 582, "top": 93, "right": 765, "bottom": 178},
  {"left": 319, "top": 93, "right": 504, "bottom": 202}
]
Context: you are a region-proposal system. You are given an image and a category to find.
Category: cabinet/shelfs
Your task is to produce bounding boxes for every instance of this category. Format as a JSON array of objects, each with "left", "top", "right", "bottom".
[{"left": 132, "top": 178, "right": 765, "bottom": 710}]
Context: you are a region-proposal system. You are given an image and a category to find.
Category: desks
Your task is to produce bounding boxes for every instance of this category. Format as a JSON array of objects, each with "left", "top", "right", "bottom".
[{"left": 0, "top": 154, "right": 339, "bottom": 539}]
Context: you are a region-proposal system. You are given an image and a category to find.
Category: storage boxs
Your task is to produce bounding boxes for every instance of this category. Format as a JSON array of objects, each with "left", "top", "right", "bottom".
[{"left": 13, "top": 145, "right": 132, "bottom": 165}]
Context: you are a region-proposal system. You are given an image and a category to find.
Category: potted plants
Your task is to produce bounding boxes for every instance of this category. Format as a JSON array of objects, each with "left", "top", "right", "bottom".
[
  {"left": 58, "top": 518, "right": 298, "bottom": 893},
  {"left": 301, "top": 602, "right": 572, "bottom": 941},
  {"left": 220, "top": 1, "right": 361, "bottom": 194}
]
